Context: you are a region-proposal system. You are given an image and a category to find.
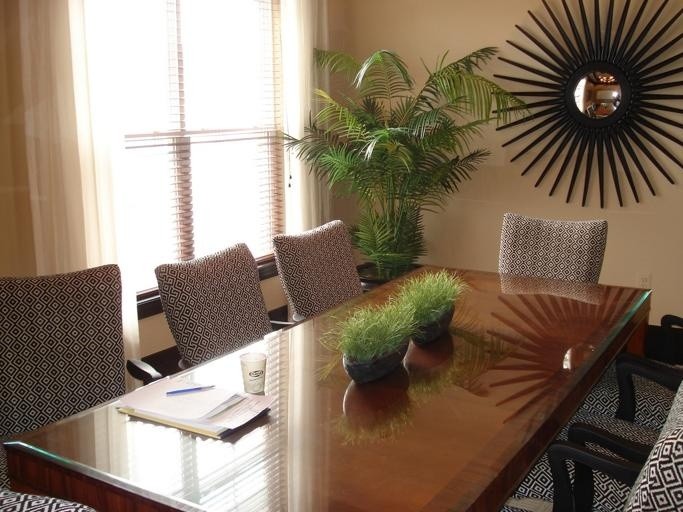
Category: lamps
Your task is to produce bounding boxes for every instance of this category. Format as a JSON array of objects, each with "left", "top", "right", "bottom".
[
  {"left": 494, "top": 314, "right": 682, "bottom": 512},
  {"left": 271, "top": 220, "right": 364, "bottom": 323},
  {"left": 1, "top": 264, "right": 163, "bottom": 512},
  {"left": 498, "top": 211, "right": 608, "bottom": 283},
  {"left": 153, "top": 243, "right": 296, "bottom": 372}
]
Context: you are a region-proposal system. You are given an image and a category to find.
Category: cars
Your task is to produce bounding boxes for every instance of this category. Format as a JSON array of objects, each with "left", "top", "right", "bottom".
[{"left": 238, "top": 350, "right": 267, "bottom": 394}]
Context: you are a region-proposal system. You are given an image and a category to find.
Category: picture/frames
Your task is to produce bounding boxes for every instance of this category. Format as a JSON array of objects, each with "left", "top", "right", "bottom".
[{"left": 3, "top": 265, "right": 652, "bottom": 511}]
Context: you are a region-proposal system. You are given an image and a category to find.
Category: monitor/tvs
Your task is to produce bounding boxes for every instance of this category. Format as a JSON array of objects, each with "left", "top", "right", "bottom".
[{"left": 116, "top": 376, "right": 278, "bottom": 440}]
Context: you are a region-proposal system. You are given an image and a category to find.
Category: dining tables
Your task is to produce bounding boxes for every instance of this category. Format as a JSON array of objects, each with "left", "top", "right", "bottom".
[
  {"left": 312, "top": 295, "right": 427, "bottom": 387},
  {"left": 336, "top": 364, "right": 427, "bottom": 446},
  {"left": 395, "top": 265, "right": 471, "bottom": 346},
  {"left": 277, "top": 46, "right": 534, "bottom": 295},
  {"left": 399, "top": 329, "right": 468, "bottom": 398}
]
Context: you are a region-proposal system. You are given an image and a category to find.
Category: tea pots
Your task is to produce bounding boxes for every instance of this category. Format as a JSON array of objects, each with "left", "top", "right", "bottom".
[
  {"left": 485, "top": 285, "right": 641, "bottom": 425},
  {"left": 489, "top": 0, "right": 683, "bottom": 209}
]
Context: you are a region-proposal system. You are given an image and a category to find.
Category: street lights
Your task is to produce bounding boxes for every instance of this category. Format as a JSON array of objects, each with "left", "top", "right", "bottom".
[{"left": 645, "top": 314, "right": 683, "bottom": 366}]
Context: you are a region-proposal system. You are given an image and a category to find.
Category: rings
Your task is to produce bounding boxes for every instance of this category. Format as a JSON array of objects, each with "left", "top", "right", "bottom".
[{"left": 165, "top": 385, "right": 216, "bottom": 399}]
